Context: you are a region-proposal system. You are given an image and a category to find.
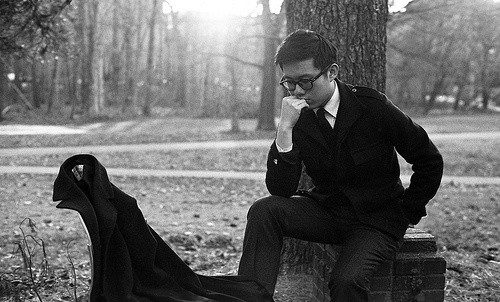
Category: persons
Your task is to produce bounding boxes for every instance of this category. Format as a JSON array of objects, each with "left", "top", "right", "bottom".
[{"left": 237, "top": 29, "right": 443, "bottom": 302}]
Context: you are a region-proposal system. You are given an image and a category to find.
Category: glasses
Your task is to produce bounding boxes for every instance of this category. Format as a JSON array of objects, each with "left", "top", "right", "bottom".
[{"left": 279, "top": 63, "right": 331, "bottom": 91}]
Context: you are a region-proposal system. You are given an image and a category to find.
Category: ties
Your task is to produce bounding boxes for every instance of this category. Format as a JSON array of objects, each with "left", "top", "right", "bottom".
[{"left": 317, "top": 108, "right": 339, "bottom": 153}]
